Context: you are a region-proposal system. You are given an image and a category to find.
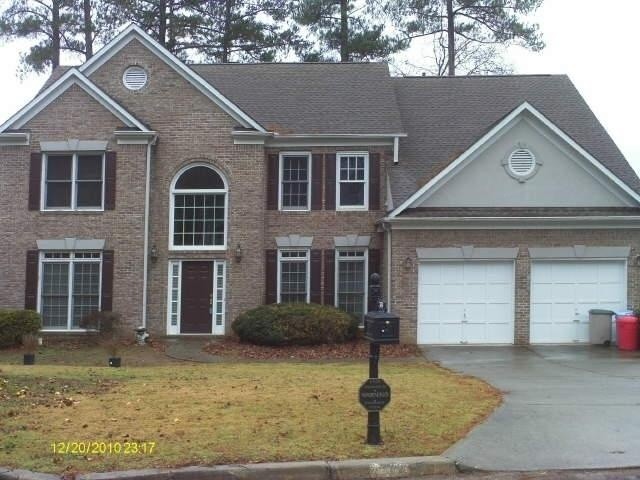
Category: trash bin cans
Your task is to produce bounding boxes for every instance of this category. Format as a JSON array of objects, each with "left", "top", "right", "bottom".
[
  {"left": 589, "top": 309, "right": 614, "bottom": 347},
  {"left": 616, "top": 314, "right": 640, "bottom": 351}
]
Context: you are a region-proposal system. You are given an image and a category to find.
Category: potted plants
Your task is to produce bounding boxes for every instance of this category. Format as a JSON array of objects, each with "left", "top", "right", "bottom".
[
  {"left": 91, "top": 333, "right": 133, "bottom": 367},
  {"left": 22, "top": 336, "right": 36, "bottom": 365}
]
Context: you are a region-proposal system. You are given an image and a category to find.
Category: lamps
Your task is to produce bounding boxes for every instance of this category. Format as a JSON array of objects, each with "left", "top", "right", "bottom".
[
  {"left": 151, "top": 246, "right": 157, "bottom": 263},
  {"left": 235, "top": 243, "right": 241, "bottom": 261}
]
